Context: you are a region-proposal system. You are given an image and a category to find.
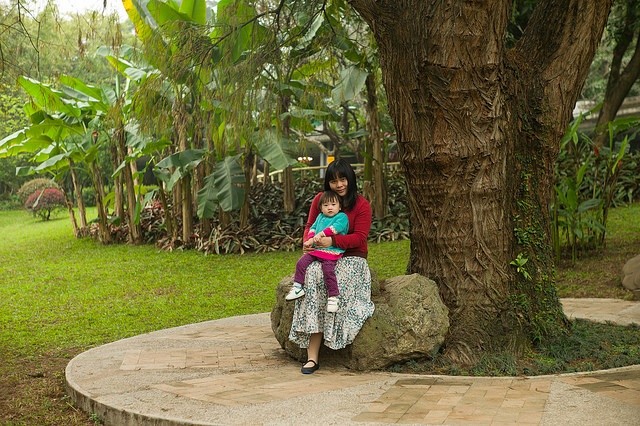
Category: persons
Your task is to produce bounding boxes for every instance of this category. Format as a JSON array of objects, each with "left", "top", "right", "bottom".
[
  {"left": 287, "top": 158, "right": 376, "bottom": 374},
  {"left": 285, "top": 191, "right": 350, "bottom": 313}
]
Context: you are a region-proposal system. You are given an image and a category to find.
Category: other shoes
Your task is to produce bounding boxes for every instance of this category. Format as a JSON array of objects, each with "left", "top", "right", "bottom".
[{"left": 301, "top": 360, "right": 319, "bottom": 374}]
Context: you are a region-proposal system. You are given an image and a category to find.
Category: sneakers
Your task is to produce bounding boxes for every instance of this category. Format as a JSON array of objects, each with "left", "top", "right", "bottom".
[
  {"left": 327, "top": 297, "right": 340, "bottom": 313},
  {"left": 286, "top": 287, "right": 305, "bottom": 302}
]
